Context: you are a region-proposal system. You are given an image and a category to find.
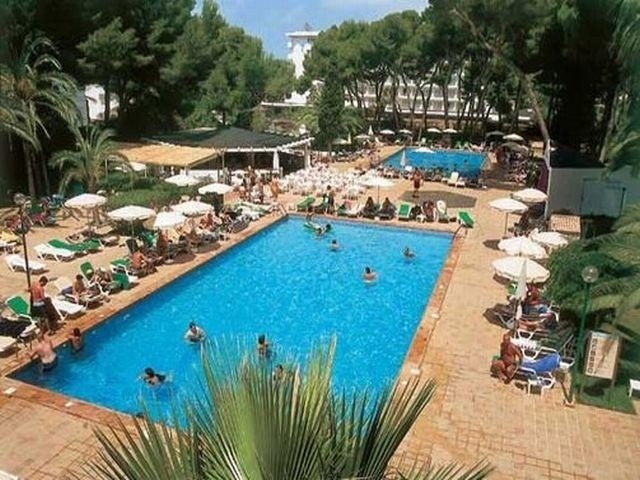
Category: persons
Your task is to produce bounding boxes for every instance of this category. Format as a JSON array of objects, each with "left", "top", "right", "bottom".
[
  {"left": 186, "top": 322, "right": 201, "bottom": 338},
  {"left": 143, "top": 367, "right": 159, "bottom": 384},
  {"left": 304, "top": 163, "right": 435, "bottom": 283},
  {"left": 28, "top": 328, "right": 57, "bottom": 369},
  {"left": 271, "top": 364, "right": 284, "bottom": 385},
  {"left": 490, "top": 282, "right": 558, "bottom": 383},
  {"left": 24, "top": 276, "right": 58, "bottom": 333},
  {"left": 66, "top": 164, "right": 285, "bottom": 302},
  {"left": 67, "top": 328, "right": 81, "bottom": 350},
  {"left": 257, "top": 335, "right": 269, "bottom": 350}
]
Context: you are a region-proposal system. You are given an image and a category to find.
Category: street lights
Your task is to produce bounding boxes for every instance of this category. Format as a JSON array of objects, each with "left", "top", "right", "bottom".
[
  {"left": 12, "top": 191, "right": 34, "bottom": 320},
  {"left": 563, "top": 264, "right": 602, "bottom": 408}
]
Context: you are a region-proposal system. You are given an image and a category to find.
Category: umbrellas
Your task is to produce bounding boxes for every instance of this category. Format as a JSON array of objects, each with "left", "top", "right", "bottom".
[
  {"left": 352, "top": 126, "right": 524, "bottom": 150},
  {"left": 487, "top": 186, "right": 570, "bottom": 300}
]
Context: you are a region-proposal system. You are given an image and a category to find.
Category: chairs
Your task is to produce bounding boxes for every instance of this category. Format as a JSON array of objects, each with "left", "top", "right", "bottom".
[
  {"left": 0, "top": 197, "right": 274, "bottom": 353},
  {"left": 491, "top": 301, "right": 576, "bottom": 398},
  {"left": 297, "top": 197, "right": 481, "bottom": 227},
  {"left": 447, "top": 171, "right": 466, "bottom": 188}
]
